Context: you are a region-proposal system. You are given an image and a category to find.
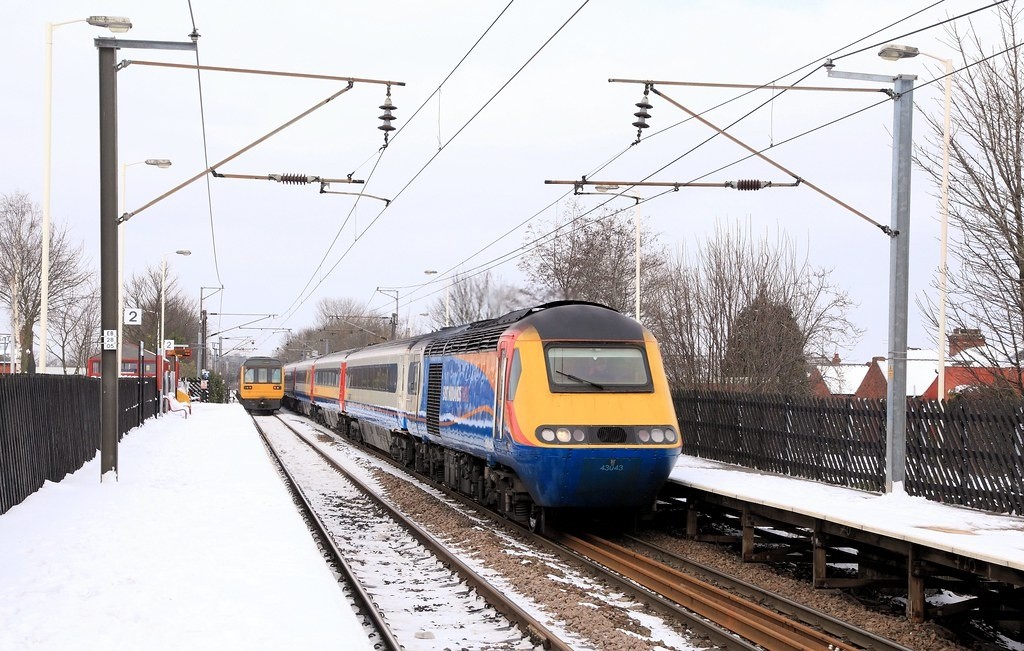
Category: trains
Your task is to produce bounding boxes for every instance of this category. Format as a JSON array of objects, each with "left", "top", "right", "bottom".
[
  {"left": 283, "top": 298, "right": 683, "bottom": 536},
  {"left": 241, "top": 356, "right": 286, "bottom": 415}
]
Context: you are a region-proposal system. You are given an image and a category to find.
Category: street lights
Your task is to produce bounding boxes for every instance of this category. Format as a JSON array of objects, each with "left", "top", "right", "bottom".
[
  {"left": 38, "top": 15, "right": 135, "bottom": 376},
  {"left": 160, "top": 248, "right": 192, "bottom": 416},
  {"left": 877, "top": 42, "right": 951, "bottom": 453},
  {"left": 424, "top": 269, "right": 449, "bottom": 325},
  {"left": 118, "top": 157, "right": 172, "bottom": 374},
  {"left": 596, "top": 183, "right": 642, "bottom": 323}
]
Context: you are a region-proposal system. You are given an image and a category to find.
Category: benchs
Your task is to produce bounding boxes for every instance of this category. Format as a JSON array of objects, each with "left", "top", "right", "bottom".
[{"left": 163, "top": 392, "right": 201, "bottom": 419}]
